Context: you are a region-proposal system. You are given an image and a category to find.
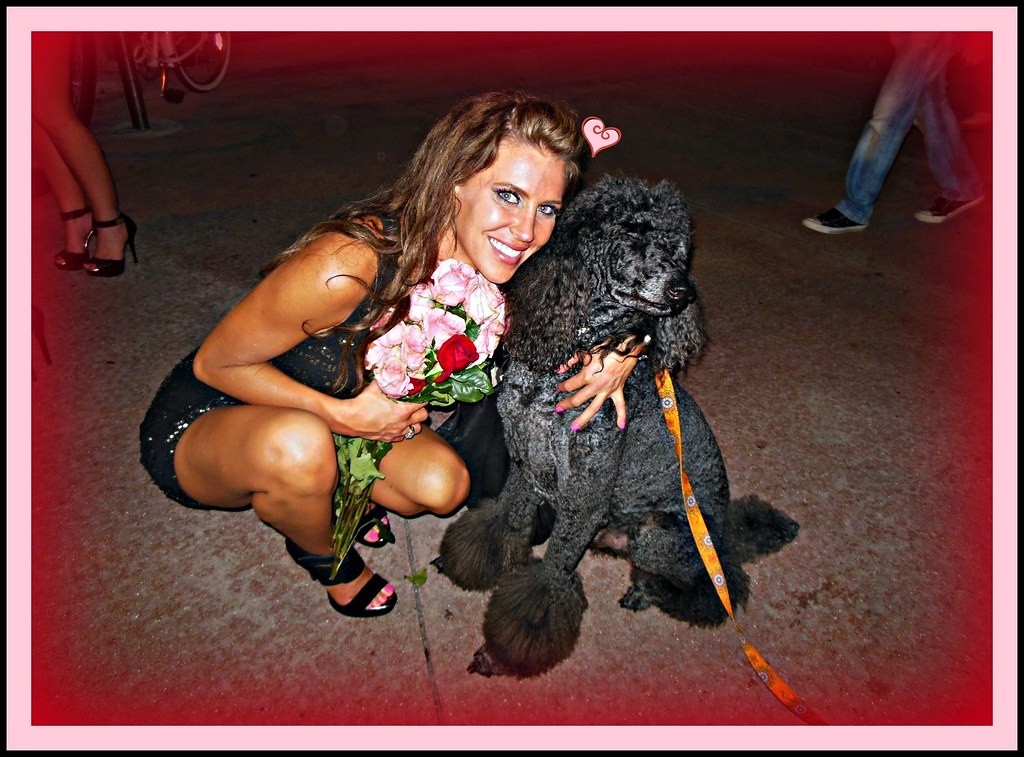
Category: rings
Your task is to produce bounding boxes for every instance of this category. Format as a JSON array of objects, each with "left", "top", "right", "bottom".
[{"left": 405, "top": 426, "right": 415, "bottom": 439}]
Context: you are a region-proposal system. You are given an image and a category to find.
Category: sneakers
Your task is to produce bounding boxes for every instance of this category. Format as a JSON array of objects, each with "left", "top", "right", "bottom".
[
  {"left": 801, "top": 207, "right": 868, "bottom": 235},
  {"left": 912, "top": 186, "right": 986, "bottom": 224}
]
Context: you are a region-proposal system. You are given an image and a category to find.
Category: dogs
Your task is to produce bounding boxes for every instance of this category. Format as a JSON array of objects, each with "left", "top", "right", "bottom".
[{"left": 428, "top": 167, "right": 800, "bottom": 680}]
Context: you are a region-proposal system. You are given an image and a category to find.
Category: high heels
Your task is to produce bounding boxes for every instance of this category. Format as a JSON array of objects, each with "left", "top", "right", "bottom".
[
  {"left": 331, "top": 498, "right": 392, "bottom": 548},
  {"left": 52, "top": 190, "right": 138, "bottom": 278},
  {"left": 284, "top": 536, "right": 398, "bottom": 618}
]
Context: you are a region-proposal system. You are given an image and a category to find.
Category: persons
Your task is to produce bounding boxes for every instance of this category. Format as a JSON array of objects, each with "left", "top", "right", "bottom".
[
  {"left": 803, "top": 32, "right": 987, "bottom": 236},
  {"left": 31, "top": 32, "right": 138, "bottom": 277},
  {"left": 139, "top": 92, "right": 645, "bottom": 618}
]
